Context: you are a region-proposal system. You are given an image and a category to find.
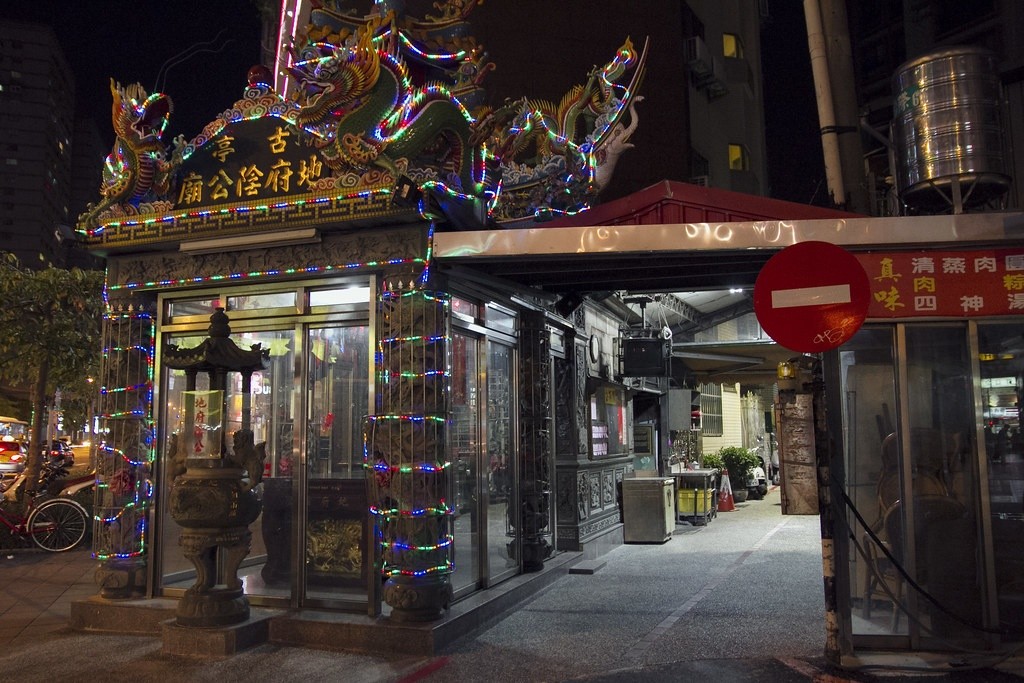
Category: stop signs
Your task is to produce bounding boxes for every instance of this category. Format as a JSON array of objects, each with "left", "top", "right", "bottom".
[{"left": 753, "top": 240, "right": 872, "bottom": 356}]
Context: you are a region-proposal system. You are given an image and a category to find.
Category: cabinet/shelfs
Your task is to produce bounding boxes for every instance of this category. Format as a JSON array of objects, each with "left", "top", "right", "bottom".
[{"left": 622, "top": 477, "right": 675, "bottom": 542}]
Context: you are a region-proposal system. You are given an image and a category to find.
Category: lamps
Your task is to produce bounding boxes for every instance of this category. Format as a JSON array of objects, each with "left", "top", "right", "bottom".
[{"left": 776, "top": 361, "right": 794, "bottom": 380}]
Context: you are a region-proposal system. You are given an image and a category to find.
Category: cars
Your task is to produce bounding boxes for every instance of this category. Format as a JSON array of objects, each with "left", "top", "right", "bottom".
[
  {"left": 0, "top": 439, "right": 28, "bottom": 480},
  {"left": 39, "top": 439, "right": 74, "bottom": 468},
  {"left": 58, "top": 436, "right": 72, "bottom": 446}
]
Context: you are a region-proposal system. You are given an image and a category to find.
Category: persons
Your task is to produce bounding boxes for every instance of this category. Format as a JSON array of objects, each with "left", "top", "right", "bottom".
[
  {"left": 244, "top": 459, "right": 261, "bottom": 491},
  {"left": 261, "top": 419, "right": 299, "bottom": 585},
  {"left": 998, "top": 423, "right": 1010, "bottom": 465}
]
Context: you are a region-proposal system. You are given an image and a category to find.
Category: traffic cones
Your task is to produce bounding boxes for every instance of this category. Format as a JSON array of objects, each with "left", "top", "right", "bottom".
[{"left": 717, "top": 470, "right": 734, "bottom": 513}]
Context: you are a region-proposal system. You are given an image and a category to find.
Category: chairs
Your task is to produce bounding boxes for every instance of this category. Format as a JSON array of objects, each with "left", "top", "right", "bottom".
[{"left": 862, "top": 390, "right": 973, "bottom": 634}]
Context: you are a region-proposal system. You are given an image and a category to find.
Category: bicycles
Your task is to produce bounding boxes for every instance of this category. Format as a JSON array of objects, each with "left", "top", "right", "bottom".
[{"left": 0, "top": 488, "right": 91, "bottom": 553}]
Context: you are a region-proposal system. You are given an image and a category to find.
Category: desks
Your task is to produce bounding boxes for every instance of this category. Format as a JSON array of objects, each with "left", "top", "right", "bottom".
[
  {"left": 669, "top": 468, "right": 717, "bottom": 526},
  {"left": 262, "top": 471, "right": 367, "bottom": 588}
]
